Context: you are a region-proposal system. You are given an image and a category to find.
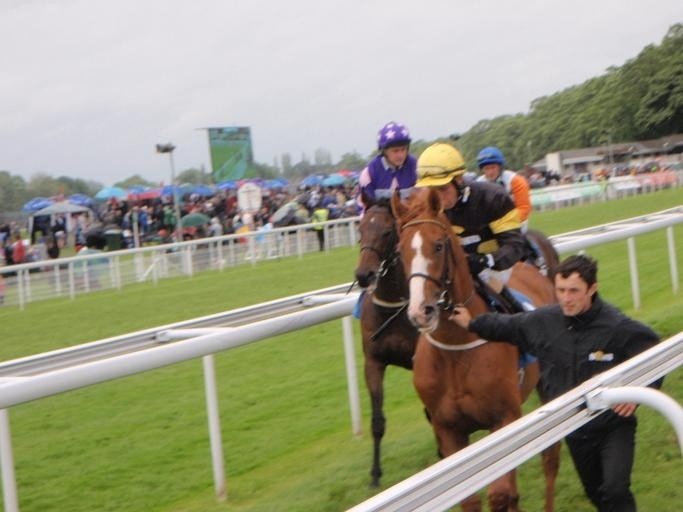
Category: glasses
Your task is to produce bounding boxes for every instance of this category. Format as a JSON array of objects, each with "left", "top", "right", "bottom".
[
  {"left": 416, "top": 165, "right": 464, "bottom": 178},
  {"left": 477, "top": 153, "right": 501, "bottom": 161}
]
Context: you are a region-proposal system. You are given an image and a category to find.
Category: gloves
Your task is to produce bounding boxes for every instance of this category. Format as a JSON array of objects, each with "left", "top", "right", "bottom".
[{"left": 464, "top": 253, "right": 489, "bottom": 274}]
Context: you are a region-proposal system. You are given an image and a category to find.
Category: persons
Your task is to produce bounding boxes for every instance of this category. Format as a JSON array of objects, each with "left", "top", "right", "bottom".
[
  {"left": 413, "top": 141, "right": 527, "bottom": 358},
  {"left": 351, "top": 119, "right": 418, "bottom": 319},
  {"left": 1, "top": 183, "right": 362, "bottom": 286},
  {"left": 474, "top": 145, "right": 548, "bottom": 277},
  {"left": 528, "top": 161, "right": 673, "bottom": 211},
  {"left": 447, "top": 254, "right": 665, "bottom": 512}
]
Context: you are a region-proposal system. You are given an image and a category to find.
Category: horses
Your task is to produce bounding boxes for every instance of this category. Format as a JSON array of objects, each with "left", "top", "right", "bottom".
[
  {"left": 355, "top": 182, "right": 561, "bottom": 491},
  {"left": 385, "top": 179, "right": 560, "bottom": 510}
]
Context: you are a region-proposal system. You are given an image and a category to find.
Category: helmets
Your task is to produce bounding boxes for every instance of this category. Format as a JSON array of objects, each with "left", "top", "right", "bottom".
[
  {"left": 414, "top": 143, "right": 465, "bottom": 187},
  {"left": 476, "top": 147, "right": 504, "bottom": 168},
  {"left": 378, "top": 122, "right": 410, "bottom": 149}
]
